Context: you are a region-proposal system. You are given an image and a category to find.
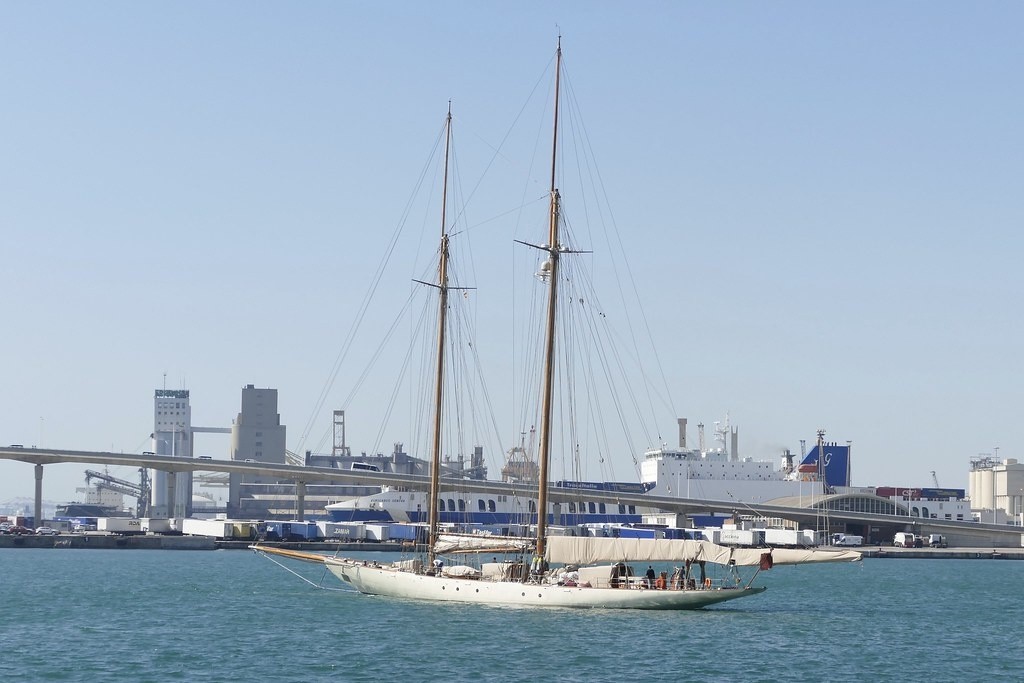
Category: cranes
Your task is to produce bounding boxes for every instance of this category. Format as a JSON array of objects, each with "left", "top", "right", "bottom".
[{"left": 83, "top": 464, "right": 152, "bottom": 516}]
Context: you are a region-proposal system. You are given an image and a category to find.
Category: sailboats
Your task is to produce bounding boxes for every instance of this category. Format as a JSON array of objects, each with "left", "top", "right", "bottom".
[{"left": 245, "top": 22, "right": 779, "bottom": 606}]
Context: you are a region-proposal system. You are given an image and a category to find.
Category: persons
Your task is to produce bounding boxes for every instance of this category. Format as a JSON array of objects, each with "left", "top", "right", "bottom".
[
  {"left": 433, "top": 560, "right": 443, "bottom": 577},
  {"left": 646, "top": 566, "right": 655, "bottom": 589},
  {"left": 492, "top": 557, "right": 497, "bottom": 563},
  {"left": 18, "top": 531, "right": 22, "bottom": 535},
  {"left": 675, "top": 565, "right": 686, "bottom": 590}
]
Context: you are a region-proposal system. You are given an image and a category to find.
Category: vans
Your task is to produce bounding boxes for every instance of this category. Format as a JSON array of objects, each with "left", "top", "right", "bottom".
[{"left": 835, "top": 536, "right": 865, "bottom": 548}]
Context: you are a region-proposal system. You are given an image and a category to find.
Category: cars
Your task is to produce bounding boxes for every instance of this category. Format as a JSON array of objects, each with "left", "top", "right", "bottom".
[
  {"left": 36, "top": 527, "right": 61, "bottom": 536},
  {"left": 0, "top": 521, "right": 36, "bottom": 536}
]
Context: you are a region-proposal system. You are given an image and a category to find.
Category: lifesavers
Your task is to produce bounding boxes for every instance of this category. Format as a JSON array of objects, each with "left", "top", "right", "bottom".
[
  {"left": 705, "top": 578, "right": 711, "bottom": 587},
  {"left": 656, "top": 579, "right": 665, "bottom": 589}
]
